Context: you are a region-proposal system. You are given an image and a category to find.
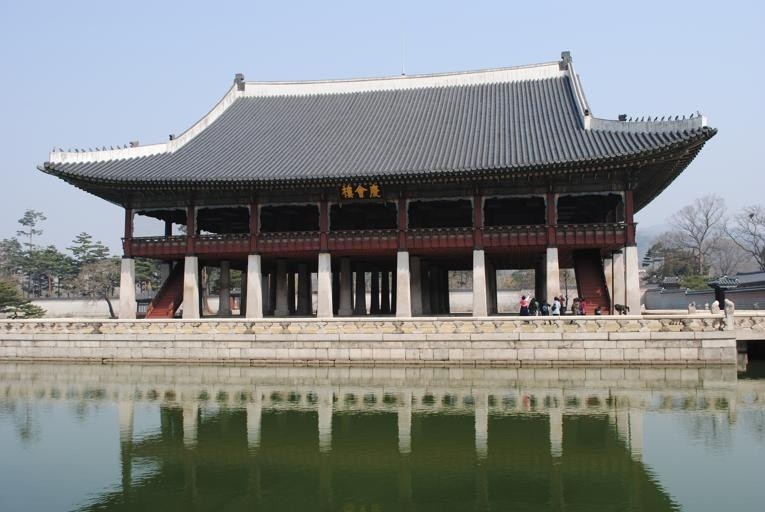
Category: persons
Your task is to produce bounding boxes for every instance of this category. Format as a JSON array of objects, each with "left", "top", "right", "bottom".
[
  {"left": 552, "top": 297, "right": 561, "bottom": 315},
  {"left": 560, "top": 293, "right": 565, "bottom": 315},
  {"left": 563, "top": 295, "right": 566, "bottom": 313},
  {"left": 595, "top": 307, "right": 601, "bottom": 314},
  {"left": 519, "top": 295, "right": 529, "bottom": 315},
  {"left": 572, "top": 296, "right": 586, "bottom": 314},
  {"left": 535, "top": 298, "right": 540, "bottom": 315},
  {"left": 538, "top": 298, "right": 550, "bottom": 315},
  {"left": 529, "top": 298, "right": 537, "bottom": 315}
]
